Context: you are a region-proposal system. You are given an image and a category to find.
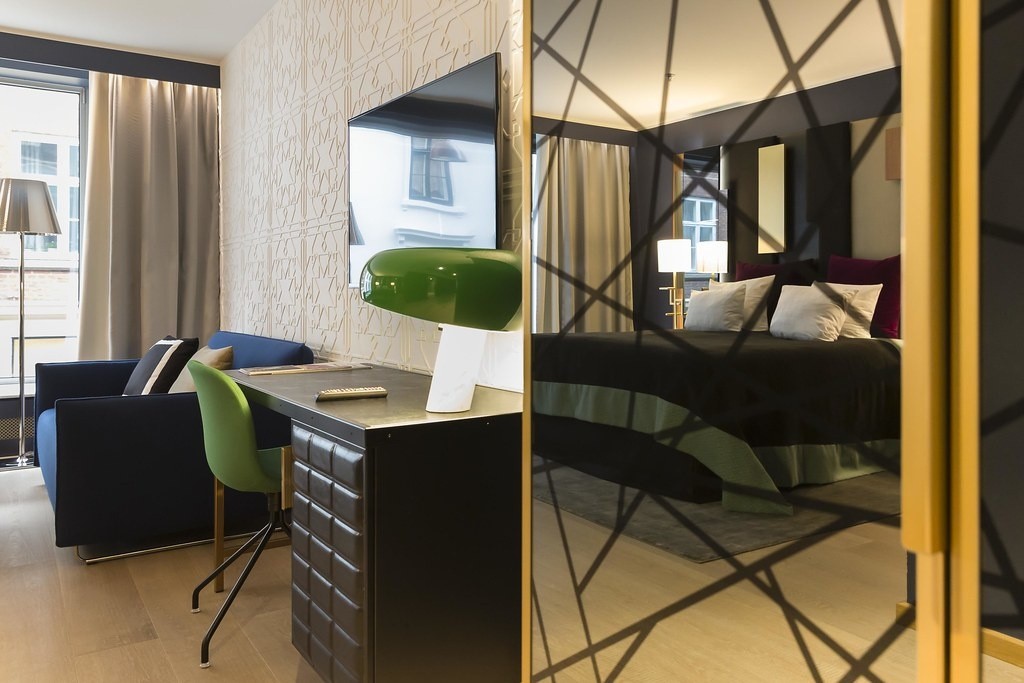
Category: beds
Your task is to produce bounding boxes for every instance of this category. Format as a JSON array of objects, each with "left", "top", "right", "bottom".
[{"left": 531, "top": 329, "right": 901, "bottom": 515}]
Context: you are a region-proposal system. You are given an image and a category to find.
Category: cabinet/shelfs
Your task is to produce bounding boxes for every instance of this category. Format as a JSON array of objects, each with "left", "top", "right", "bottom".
[{"left": 522, "top": 0, "right": 1024, "bottom": 683}]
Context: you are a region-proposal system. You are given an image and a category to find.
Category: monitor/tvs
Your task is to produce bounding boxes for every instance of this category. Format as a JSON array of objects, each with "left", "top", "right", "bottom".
[{"left": 344, "top": 52, "right": 500, "bottom": 288}]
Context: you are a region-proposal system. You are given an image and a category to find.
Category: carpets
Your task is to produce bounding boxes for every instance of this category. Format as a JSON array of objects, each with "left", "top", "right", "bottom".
[{"left": 532, "top": 455, "right": 900, "bottom": 565}]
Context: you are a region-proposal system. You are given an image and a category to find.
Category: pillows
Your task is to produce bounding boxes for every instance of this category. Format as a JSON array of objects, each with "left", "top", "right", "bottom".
[
  {"left": 684, "top": 284, "right": 746, "bottom": 332},
  {"left": 168, "top": 345, "right": 233, "bottom": 394},
  {"left": 811, "top": 281, "right": 883, "bottom": 339},
  {"left": 770, "top": 285, "right": 858, "bottom": 343},
  {"left": 735, "top": 258, "right": 816, "bottom": 320},
  {"left": 708, "top": 275, "right": 776, "bottom": 331},
  {"left": 122, "top": 335, "right": 199, "bottom": 396},
  {"left": 827, "top": 254, "right": 901, "bottom": 339}
]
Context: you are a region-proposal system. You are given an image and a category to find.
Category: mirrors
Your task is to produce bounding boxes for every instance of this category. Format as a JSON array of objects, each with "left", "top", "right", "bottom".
[
  {"left": 532, "top": 0, "right": 916, "bottom": 683},
  {"left": 682, "top": 146, "right": 720, "bottom": 324}
]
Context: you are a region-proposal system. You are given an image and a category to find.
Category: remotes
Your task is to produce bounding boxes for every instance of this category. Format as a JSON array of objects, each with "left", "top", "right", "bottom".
[{"left": 314, "top": 387, "right": 388, "bottom": 402}]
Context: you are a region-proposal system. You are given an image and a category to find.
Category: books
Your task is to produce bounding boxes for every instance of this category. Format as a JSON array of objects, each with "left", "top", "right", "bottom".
[{"left": 238, "top": 361, "right": 353, "bottom": 376}]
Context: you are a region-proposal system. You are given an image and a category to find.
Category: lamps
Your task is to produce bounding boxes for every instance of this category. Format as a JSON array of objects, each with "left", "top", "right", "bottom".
[
  {"left": 357, "top": 248, "right": 522, "bottom": 332},
  {"left": 657, "top": 239, "right": 728, "bottom": 329},
  {"left": 0, "top": 179, "right": 62, "bottom": 473}
]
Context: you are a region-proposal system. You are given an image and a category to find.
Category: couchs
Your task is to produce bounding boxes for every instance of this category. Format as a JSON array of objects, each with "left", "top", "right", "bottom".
[{"left": 34, "top": 332, "right": 314, "bottom": 565}]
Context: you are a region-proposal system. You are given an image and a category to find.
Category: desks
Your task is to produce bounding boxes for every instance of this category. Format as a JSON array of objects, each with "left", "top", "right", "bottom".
[{"left": 213, "top": 363, "right": 523, "bottom": 683}]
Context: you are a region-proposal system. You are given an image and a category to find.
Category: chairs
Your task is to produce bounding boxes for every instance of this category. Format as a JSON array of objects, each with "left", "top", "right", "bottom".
[{"left": 186, "top": 360, "right": 292, "bottom": 669}]
